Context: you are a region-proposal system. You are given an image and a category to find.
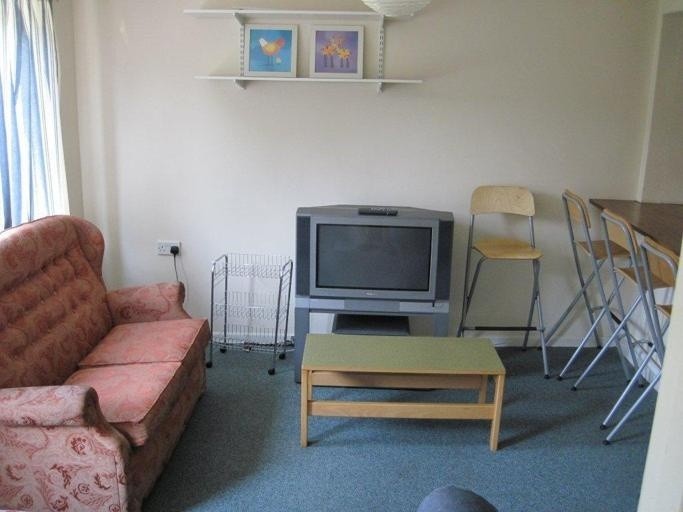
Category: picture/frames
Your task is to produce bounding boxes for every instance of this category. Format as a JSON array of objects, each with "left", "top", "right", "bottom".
[
  {"left": 243, "top": 24, "right": 298, "bottom": 77},
  {"left": 310, "top": 24, "right": 365, "bottom": 79}
]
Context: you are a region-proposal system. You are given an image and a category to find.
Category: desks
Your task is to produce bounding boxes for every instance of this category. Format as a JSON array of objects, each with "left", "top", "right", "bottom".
[{"left": 589, "top": 197, "right": 683, "bottom": 258}]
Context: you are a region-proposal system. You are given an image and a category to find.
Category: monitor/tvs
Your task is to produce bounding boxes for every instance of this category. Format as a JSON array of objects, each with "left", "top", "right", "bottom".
[{"left": 294, "top": 204, "right": 455, "bottom": 315}]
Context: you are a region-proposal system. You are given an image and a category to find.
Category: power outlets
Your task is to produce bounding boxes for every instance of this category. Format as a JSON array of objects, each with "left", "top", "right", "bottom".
[{"left": 156, "top": 240, "right": 179, "bottom": 256}]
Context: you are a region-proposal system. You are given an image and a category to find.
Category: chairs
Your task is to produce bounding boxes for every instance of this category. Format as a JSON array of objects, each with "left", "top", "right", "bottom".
[
  {"left": 569, "top": 208, "right": 662, "bottom": 430},
  {"left": 538, "top": 189, "right": 632, "bottom": 383},
  {"left": 604, "top": 236, "right": 683, "bottom": 446},
  {"left": 457, "top": 185, "right": 549, "bottom": 379}
]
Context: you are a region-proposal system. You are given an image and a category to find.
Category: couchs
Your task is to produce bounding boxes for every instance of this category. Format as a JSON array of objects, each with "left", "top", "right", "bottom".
[{"left": 0, "top": 214, "right": 209, "bottom": 512}]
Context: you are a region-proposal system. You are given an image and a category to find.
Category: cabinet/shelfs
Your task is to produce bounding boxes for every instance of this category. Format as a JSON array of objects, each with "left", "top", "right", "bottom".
[
  {"left": 182, "top": 9, "right": 424, "bottom": 96},
  {"left": 294, "top": 306, "right": 449, "bottom": 384}
]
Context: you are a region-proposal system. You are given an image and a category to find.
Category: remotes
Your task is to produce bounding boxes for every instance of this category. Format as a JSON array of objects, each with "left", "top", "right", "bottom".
[{"left": 358, "top": 206, "right": 398, "bottom": 216}]
